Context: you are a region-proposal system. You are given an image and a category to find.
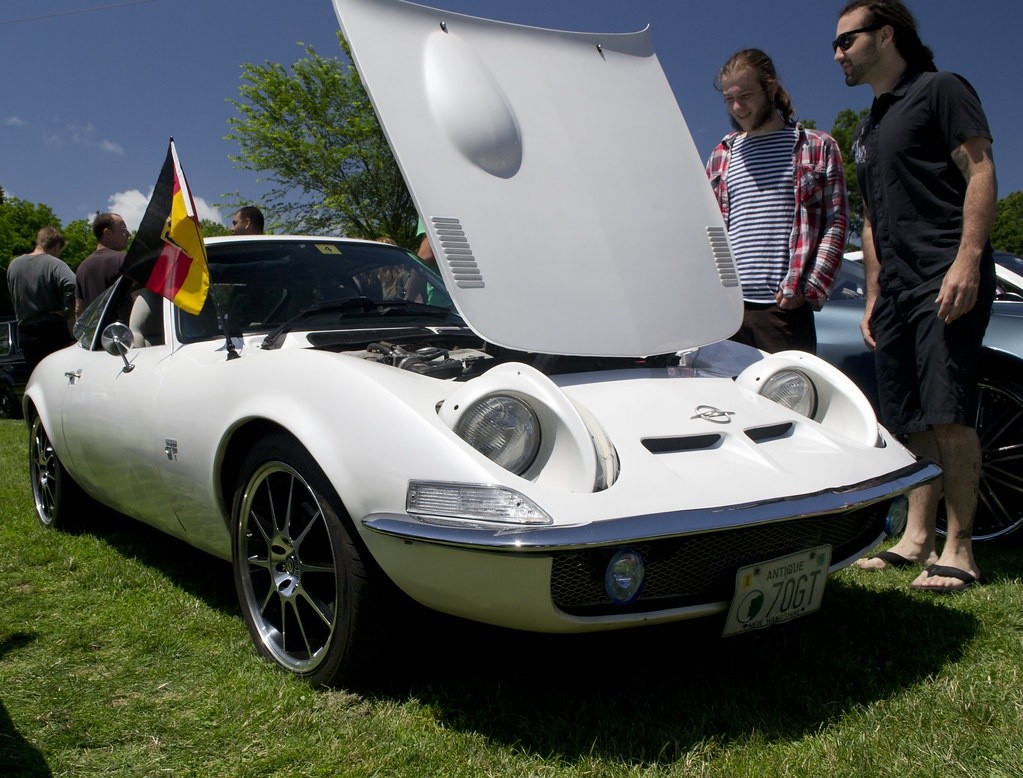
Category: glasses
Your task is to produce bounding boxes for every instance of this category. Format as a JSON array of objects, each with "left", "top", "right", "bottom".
[{"left": 833, "top": 24, "right": 881, "bottom": 53}]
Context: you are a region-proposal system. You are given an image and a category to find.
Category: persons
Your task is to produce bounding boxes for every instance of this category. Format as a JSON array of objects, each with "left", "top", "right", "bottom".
[
  {"left": 229, "top": 206, "right": 264, "bottom": 236},
  {"left": 344, "top": 236, "right": 421, "bottom": 304},
  {"left": 703, "top": 47, "right": 846, "bottom": 353},
  {"left": 7, "top": 226, "right": 76, "bottom": 374},
  {"left": 415, "top": 215, "right": 455, "bottom": 307},
  {"left": 834, "top": 0, "right": 998, "bottom": 591},
  {"left": 76, "top": 212, "right": 132, "bottom": 311}
]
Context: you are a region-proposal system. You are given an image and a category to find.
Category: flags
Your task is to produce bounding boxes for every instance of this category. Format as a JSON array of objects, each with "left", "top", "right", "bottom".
[{"left": 118, "top": 136, "right": 208, "bottom": 317}]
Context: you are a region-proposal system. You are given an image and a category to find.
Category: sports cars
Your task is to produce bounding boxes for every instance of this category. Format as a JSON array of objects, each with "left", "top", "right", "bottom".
[{"left": 21, "top": 0, "right": 946, "bottom": 689}]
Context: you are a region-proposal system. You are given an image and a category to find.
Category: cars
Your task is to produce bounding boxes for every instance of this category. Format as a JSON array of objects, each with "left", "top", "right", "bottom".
[
  {"left": 841, "top": 251, "right": 1023, "bottom": 300},
  {"left": 819, "top": 254, "right": 1023, "bottom": 560}
]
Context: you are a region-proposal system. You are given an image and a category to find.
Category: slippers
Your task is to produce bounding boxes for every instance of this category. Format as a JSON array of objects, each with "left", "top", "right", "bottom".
[
  {"left": 853, "top": 544, "right": 936, "bottom": 572},
  {"left": 909, "top": 558, "right": 986, "bottom": 593}
]
